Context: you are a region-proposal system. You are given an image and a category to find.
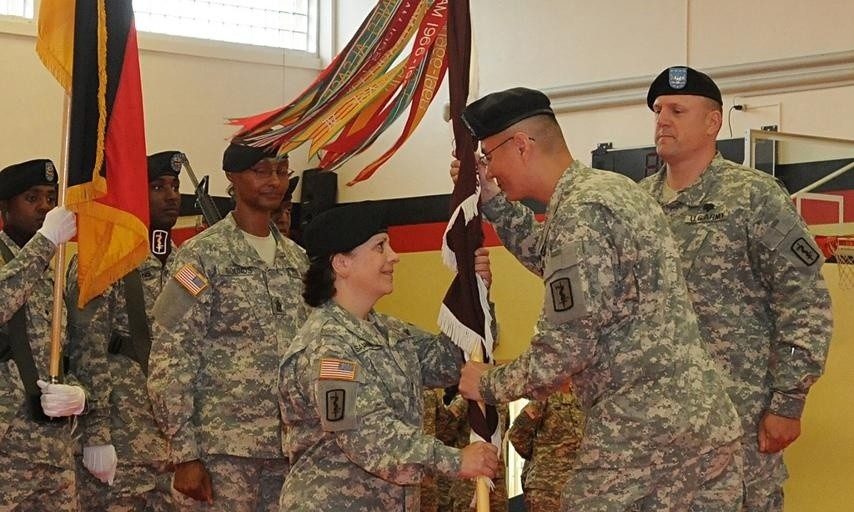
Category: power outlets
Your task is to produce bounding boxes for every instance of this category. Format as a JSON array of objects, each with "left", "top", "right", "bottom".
[{"left": 735, "top": 104, "right": 747, "bottom": 112}]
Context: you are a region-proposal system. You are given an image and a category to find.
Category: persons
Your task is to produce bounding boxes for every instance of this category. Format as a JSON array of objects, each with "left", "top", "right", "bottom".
[
  {"left": 1, "top": 159, "right": 92, "bottom": 511},
  {"left": 637, "top": 67, "right": 834, "bottom": 512},
  {"left": 146, "top": 126, "right": 318, "bottom": 512},
  {"left": 274, "top": 174, "right": 299, "bottom": 236},
  {"left": 457, "top": 88, "right": 749, "bottom": 512},
  {"left": 276, "top": 201, "right": 499, "bottom": 511},
  {"left": 70, "top": 148, "right": 186, "bottom": 511}
]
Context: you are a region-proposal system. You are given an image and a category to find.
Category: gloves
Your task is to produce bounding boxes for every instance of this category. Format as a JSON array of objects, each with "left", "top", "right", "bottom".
[
  {"left": 37, "top": 379, "right": 86, "bottom": 418},
  {"left": 82, "top": 446, "right": 118, "bottom": 487},
  {"left": 38, "top": 207, "right": 78, "bottom": 247}
]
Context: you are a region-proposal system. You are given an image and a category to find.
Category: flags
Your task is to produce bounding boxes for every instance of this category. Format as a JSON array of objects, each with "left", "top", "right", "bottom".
[{"left": 35, "top": 0, "right": 151, "bottom": 310}]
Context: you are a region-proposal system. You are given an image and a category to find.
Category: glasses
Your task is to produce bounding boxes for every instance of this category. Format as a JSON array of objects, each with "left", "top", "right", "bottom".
[
  {"left": 253, "top": 165, "right": 292, "bottom": 179},
  {"left": 479, "top": 134, "right": 535, "bottom": 167}
]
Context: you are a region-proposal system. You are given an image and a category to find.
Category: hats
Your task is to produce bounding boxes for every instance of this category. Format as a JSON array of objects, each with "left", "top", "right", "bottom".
[
  {"left": 1, "top": 159, "right": 58, "bottom": 199},
  {"left": 646, "top": 66, "right": 723, "bottom": 113},
  {"left": 222, "top": 130, "right": 289, "bottom": 173},
  {"left": 458, "top": 88, "right": 553, "bottom": 142},
  {"left": 303, "top": 200, "right": 396, "bottom": 253},
  {"left": 148, "top": 151, "right": 182, "bottom": 180}
]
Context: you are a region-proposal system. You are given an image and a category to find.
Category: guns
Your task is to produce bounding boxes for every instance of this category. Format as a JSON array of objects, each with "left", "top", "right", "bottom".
[{"left": 178, "top": 152, "right": 223, "bottom": 227}]
